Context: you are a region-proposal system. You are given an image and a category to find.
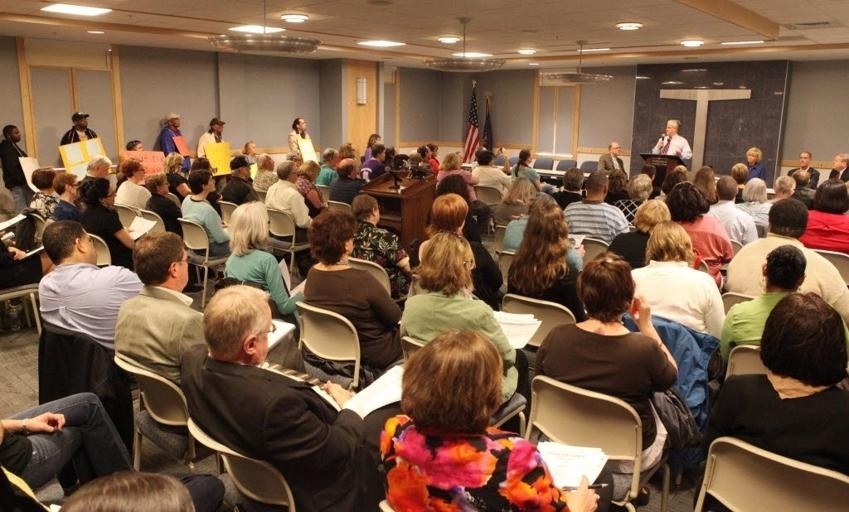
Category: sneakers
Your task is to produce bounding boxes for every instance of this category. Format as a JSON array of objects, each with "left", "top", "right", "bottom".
[{"left": 633, "top": 486, "right": 649, "bottom": 504}]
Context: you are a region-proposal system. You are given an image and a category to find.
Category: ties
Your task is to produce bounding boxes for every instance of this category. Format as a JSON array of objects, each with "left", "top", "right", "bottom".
[{"left": 663, "top": 138, "right": 671, "bottom": 155}]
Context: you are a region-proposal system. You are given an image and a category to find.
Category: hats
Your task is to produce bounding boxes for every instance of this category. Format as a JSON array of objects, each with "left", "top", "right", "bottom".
[
  {"left": 164, "top": 112, "right": 180, "bottom": 125},
  {"left": 230, "top": 156, "right": 255, "bottom": 170},
  {"left": 210, "top": 118, "right": 225, "bottom": 126},
  {"left": 72, "top": 112, "right": 90, "bottom": 121}
]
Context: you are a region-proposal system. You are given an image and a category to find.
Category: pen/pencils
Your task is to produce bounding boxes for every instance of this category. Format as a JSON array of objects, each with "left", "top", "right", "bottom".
[{"left": 562, "top": 483, "right": 609, "bottom": 491}]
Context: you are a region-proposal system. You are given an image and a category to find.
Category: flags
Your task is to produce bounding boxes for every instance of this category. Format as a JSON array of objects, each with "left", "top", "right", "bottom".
[{"left": 464, "top": 86, "right": 492, "bottom": 165}]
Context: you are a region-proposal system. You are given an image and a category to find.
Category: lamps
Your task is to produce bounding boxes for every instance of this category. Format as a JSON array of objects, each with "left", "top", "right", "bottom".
[
  {"left": 356, "top": 77, "right": 366, "bottom": 104},
  {"left": 538, "top": 40, "right": 613, "bottom": 84},
  {"left": 207, "top": 0, "right": 320, "bottom": 54},
  {"left": 421, "top": 17, "right": 506, "bottom": 73}
]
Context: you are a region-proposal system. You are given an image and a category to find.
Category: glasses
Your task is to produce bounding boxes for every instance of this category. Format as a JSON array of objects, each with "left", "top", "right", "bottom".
[
  {"left": 178, "top": 256, "right": 191, "bottom": 264},
  {"left": 262, "top": 323, "right": 276, "bottom": 334}
]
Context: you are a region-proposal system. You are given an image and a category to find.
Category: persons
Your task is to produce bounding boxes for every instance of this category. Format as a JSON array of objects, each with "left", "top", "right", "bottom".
[{"left": 1, "top": 110, "right": 849, "bottom": 511}]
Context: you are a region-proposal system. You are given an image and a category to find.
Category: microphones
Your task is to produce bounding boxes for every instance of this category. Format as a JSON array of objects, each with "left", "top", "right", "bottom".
[
  {"left": 661, "top": 134, "right": 665, "bottom": 148},
  {"left": 384, "top": 165, "right": 403, "bottom": 184}
]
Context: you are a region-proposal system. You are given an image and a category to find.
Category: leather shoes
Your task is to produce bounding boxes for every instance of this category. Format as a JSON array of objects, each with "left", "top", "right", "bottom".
[{"left": 183, "top": 285, "right": 203, "bottom": 292}]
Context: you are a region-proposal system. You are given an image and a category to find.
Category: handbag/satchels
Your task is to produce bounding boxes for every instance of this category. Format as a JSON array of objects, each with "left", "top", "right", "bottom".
[{"left": 8, "top": 301, "right": 26, "bottom": 332}]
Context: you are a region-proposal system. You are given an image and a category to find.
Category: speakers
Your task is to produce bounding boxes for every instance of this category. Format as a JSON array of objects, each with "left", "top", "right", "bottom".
[{"left": 356, "top": 78, "right": 368, "bottom": 105}]
